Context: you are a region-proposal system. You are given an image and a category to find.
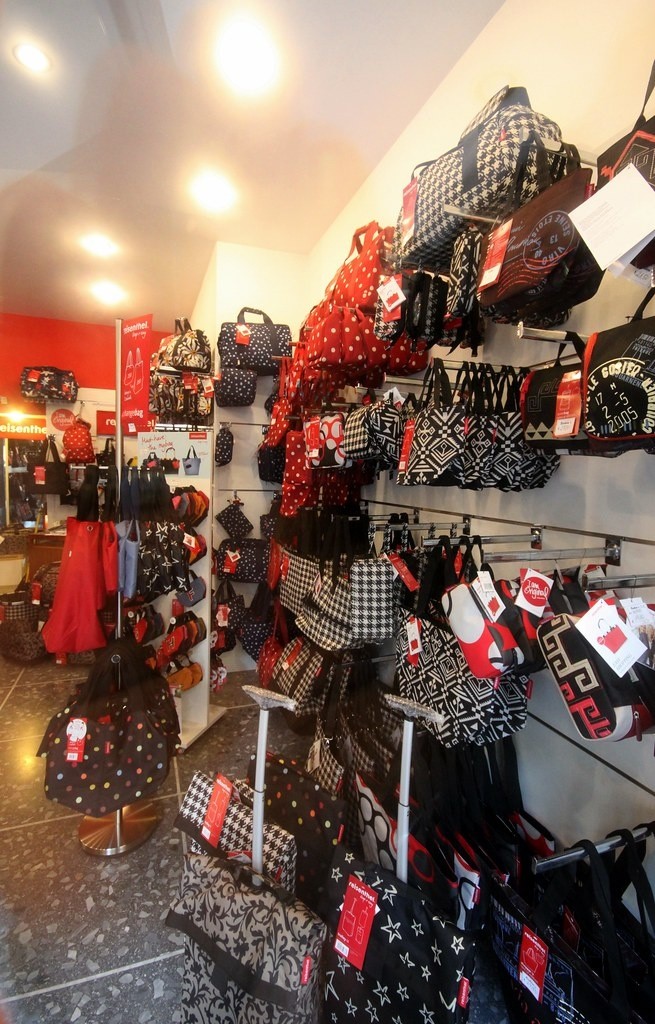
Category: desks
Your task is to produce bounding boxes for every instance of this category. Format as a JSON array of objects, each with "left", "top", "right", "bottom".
[{"left": 28, "top": 530, "right": 66, "bottom": 584}]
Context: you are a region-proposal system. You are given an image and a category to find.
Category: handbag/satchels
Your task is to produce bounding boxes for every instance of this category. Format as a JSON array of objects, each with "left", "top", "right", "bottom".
[
  {"left": 36, "top": 638, "right": 181, "bottom": 817},
  {"left": 96, "top": 438, "right": 116, "bottom": 479},
  {"left": 148, "top": 316, "right": 214, "bottom": 423},
  {"left": 62, "top": 415, "right": 95, "bottom": 463},
  {"left": 27, "top": 439, "right": 70, "bottom": 494},
  {"left": 165, "top": 86, "right": 655, "bottom": 1024},
  {"left": 118, "top": 465, "right": 206, "bottom": 692},
  {"left": 143, "top": 446, "right": 200, "bottom": 475},
  {"left": 0, "top": 592, "right": 48, "bottom": 661}
]
